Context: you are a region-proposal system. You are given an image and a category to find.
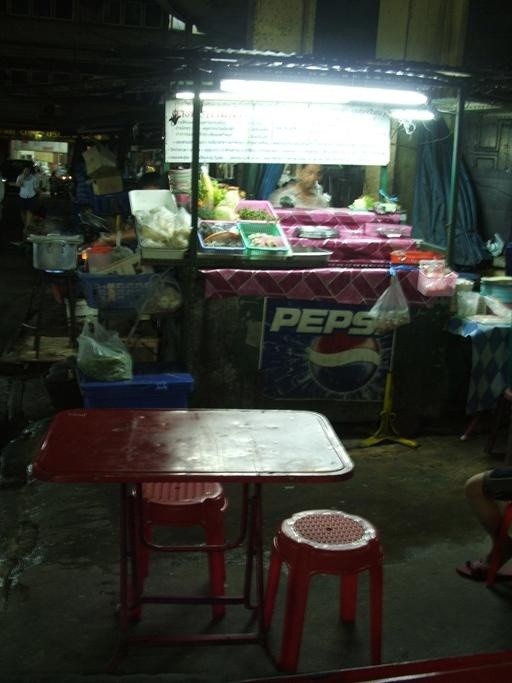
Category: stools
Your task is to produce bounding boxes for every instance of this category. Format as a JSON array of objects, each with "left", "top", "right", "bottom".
[
  {"left": 123, "top": 482, "right": 228, "bottom": 621},
  {"left": 261, "top": 509, "right": 384, "bottom": 672},
  {"left": 7, "top": 270, "right": 79, "bottom": 359},
  {"left": 459, "top": 385, "right": 512, "bottom": 466},
  {"left": 98, "top": 308, "right": 163, "bottom": 361}
]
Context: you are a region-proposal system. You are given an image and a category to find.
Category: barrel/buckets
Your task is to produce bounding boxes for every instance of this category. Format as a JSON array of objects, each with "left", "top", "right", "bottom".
[{"left": 390, "top": 251, "right": 446, "bottom": 303}]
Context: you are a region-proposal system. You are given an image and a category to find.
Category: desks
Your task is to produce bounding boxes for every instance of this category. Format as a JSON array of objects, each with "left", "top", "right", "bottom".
[{"left": 33, "top": 407, "right": 356, "bottom": 644}]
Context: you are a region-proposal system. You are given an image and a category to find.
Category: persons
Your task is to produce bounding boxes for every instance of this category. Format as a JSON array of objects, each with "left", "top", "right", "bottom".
[
  {"left": 454, "top": 465, "right": 512, "bottom": 583},
  {"left": 266, "top": 164, "right": 331, "bottom": 209},
  {"left": 0, "top": 155, "right": 166, "bottom": 274}
]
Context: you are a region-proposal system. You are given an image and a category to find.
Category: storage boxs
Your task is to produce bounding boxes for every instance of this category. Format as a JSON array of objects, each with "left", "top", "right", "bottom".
[
  {"left": 82, "top": 143, "right": 124, "bottom": 196},
  {"left": 75, "top": 361, "right": 194, "bottom": 408}
]
